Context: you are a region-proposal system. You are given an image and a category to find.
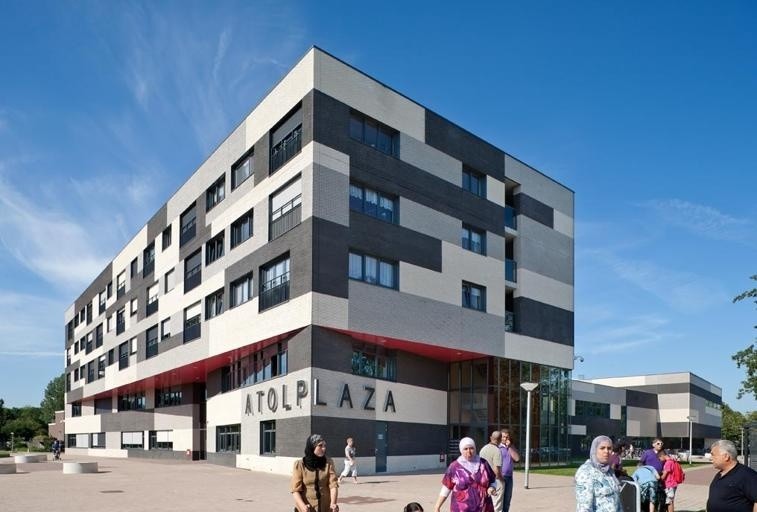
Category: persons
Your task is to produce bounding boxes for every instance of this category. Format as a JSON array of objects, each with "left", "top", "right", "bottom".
[
  {"left": 338, "top": 437, "right": 359, "bottom": 484},
  {"left": 52, "top": 440, "right": 58, "bottom": 454},
  {"left": 434, "top": 429, "right": 521, "bottom": 512},
  {"left": 706, "top": 439, "right": 757, "bottom": 512},
  {"left": 575, "top": 435, "right": 686, "bottom": 512},
  {"left": 290, "top": 434, "right": 339, "bottom": 512},
  {"left": 404, "top": 502, "right": 424, "bottom": 512}
]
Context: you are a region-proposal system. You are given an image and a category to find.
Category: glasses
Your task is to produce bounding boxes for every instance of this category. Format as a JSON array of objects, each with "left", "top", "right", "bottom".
[{"left": 657, "top": 443, "right": 663, "bottom": 445}]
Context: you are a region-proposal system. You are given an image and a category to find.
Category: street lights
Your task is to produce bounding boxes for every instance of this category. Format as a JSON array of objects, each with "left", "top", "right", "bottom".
[
  {"left": 10, "top": 432, "right": 14, "bottom": 452},
  {"left": 687, "top": 415, "right": 696, "bottom": 465},
  {"left": 740, "top": 427, "right": 745, "bottom": 459},
  {"left": 520, "top": 381, "right": 539, "bottom": 489},
  {"left": 573, "top": 355, "right": 584, "bottom": 371}
]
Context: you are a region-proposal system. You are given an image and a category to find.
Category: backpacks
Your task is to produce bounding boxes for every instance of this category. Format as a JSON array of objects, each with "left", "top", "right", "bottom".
[{"left": 668, "top": 460, "right": 685, "bottom": 483}]
